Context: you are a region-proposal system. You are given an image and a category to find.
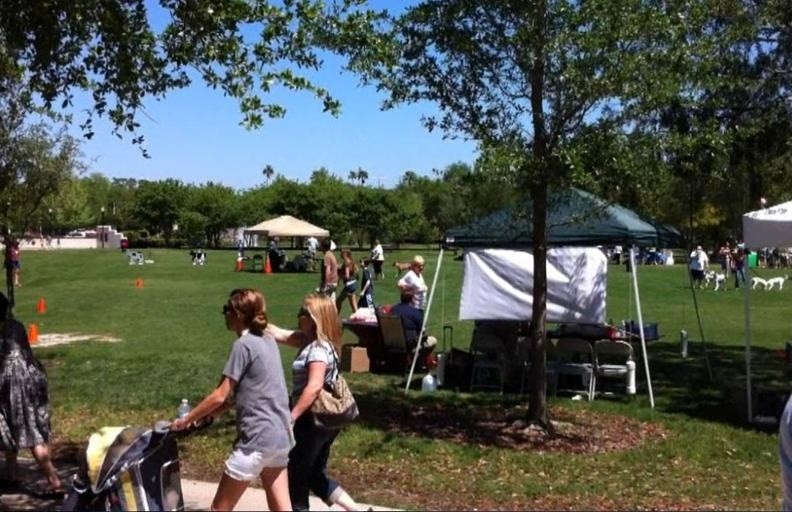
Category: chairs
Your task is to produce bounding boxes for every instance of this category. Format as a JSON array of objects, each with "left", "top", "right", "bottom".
[
  {"left": 551, "top": 336, "right": 593, "bottom": 402},
  {"left": 592, "top": 338, "right": 636, "bottom": 399},
  {"left": 509, "top": 337, "right": 558, "bottom": 397},
  {"left": 468, "top": 336, "right": 509, "bottom": 396},
  {"left": 374, "top": 311, "right": 428, "bottom": 372}
]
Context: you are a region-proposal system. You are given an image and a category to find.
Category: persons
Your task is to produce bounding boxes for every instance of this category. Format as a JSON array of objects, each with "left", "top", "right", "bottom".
[
  {"left": 777, "top": 392, "right": 792, "bottom": 512},
  {"left": 321, "top": 236, "right": 385, "bottom": 315},
  {"left": 607, "top": 244, "right": 663, "bottom": 269},
  {"left": 171, "top": 289, "right": 297, "bottom": 512},
  {"left": 191, "top": 249, "right": 205, "bottom": 265},
  {"left": 691, "top": 241, "right": 792, "bottom": 289},
  {"left": 264, "top": 293, "right": 374, "bottom": 512},
  {"left": 393, "top": 254, "right": 437, "bottom": 356},
  {"left": 9, "top": 236, "right": 21, "bottom": 285},
  {"left": 268, "top": 236, "right": 318, "bottom": 272},
  {"left": 1, "top": 291, "right": 68, "bottom": 500}
]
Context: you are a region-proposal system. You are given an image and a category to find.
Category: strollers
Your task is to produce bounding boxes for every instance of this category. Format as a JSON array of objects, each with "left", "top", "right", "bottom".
[{"left": 61, "top": 415, "right": 214, "bottom": 512}]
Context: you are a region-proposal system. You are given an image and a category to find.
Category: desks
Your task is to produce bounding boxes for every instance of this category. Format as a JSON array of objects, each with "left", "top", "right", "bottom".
[{"left": 341, "top": 314, "right": 381, "bottom": 332}]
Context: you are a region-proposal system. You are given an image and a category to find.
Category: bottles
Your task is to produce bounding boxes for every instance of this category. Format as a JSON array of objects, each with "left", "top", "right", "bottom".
[
  {"left": 436, "top": 354, "right": 441, "bottom": 365},
  {"left": 680, "top": 328, "right": 691, "bottom": 357},
  {"left": 178, "top": 396, "right": 192, "bottom": 424},
  {"left": 422, "top": 372, "right": 436, "bottom": 393},
  {"left": 606, "top": 319, "right": 619, "bottom": 340},
  {"left": 619, "top": 318, "right": 628, "bottom": 338}
]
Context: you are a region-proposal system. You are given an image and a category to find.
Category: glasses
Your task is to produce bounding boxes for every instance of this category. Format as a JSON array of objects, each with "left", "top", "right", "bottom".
[{"left": 221, "top": 305, "right": 227, "bottom": 314}]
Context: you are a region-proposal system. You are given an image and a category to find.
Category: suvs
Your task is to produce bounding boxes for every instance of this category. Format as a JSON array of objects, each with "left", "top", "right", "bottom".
[{"left": 63, "top": 228, "right": 97, "bottom": 238}]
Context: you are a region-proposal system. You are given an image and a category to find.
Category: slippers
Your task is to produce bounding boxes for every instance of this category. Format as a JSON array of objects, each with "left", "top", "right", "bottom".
[{"left": 32, "top": 489, "right": 65, "bottom": 499}]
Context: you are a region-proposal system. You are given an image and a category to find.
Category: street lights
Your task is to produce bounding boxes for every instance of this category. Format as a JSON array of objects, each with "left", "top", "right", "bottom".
[
  {"left": 49, "top": 208, "right": 53, "bottom": 236},
  {"left": 102, "top": 206, "right": 105, "bottom": 248}
]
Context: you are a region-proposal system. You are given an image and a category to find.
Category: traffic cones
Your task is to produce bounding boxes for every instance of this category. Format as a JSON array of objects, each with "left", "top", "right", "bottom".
[
  {"left": 264, "top": 256, "right": 273, "bottom": 273},
  {"left": 36, "top": 297, "right": 47, "bottom": 313},
  {"left": 27, "top": 323, "right": 40, "bottom": 345},
  {"left": 135, "top": 278, "right": 143, "bottom": 288}
]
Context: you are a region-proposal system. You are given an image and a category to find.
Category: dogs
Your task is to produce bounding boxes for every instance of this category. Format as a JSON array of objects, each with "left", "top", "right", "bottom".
[
  {"left": 392, "top": 261, "right": 413, "bottom": 278},
  {"left": 766, "top": 273, "right": 788, "bottom": 291},
  {"left": 703, "top": 270, "right": 715, "bottom": 289},
  {"left": 713, "top": 272, "right": 727, "bottom": 292},
  {"left": 750, "top": 277, "right": 766, "bottom": 291}
]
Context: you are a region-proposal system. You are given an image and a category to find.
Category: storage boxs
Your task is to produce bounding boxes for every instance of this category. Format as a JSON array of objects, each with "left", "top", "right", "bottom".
[{"left": 341, "top": 344, "right": 376, "bottom": 376}]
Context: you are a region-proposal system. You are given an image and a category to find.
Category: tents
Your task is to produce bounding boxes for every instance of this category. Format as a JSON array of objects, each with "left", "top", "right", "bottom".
[{"left": 244, "top": 213, "right": 329, "bottom": 265}]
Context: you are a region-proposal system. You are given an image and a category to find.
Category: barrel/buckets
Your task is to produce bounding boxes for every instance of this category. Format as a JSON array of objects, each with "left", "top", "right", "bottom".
[{"left": 421, "top": 369, "right": 437, "bottom": 392}]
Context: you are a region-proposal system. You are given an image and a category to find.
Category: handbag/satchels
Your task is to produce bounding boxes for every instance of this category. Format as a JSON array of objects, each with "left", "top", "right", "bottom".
[{"left": 306, "top": 340, "right": 360, "bottom": 432}]
